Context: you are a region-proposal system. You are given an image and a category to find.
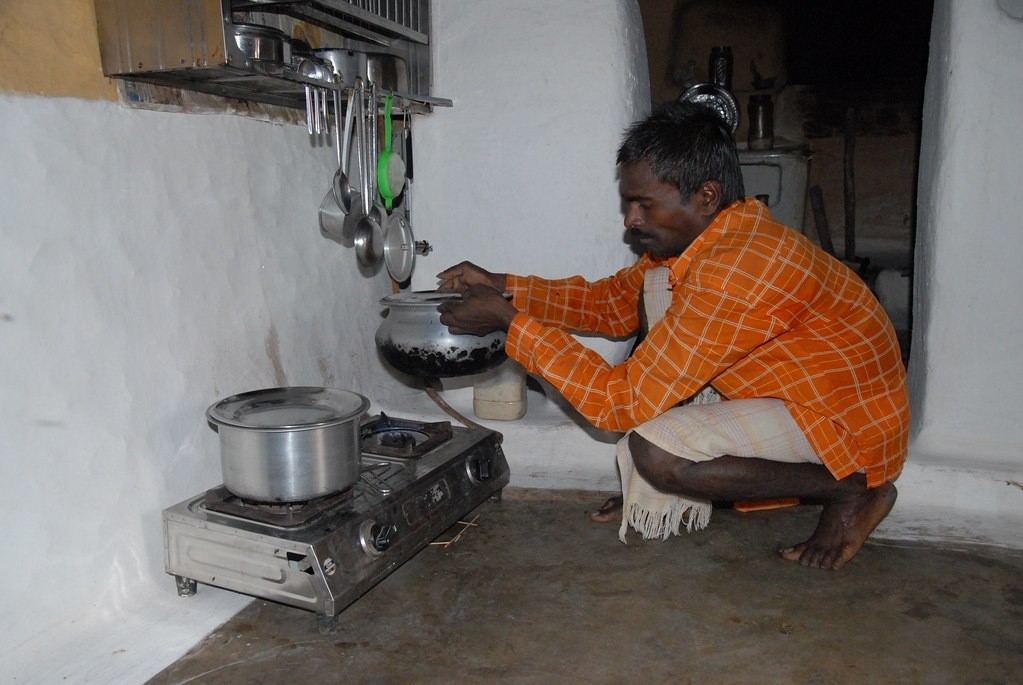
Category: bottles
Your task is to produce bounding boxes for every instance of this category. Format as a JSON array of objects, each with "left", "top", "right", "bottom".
[
  {"left": 747, "top": 93, "right": 774, "bottom": 151},
  {"left": 709, "top": 46, "right": 733, "bottom": 89}
]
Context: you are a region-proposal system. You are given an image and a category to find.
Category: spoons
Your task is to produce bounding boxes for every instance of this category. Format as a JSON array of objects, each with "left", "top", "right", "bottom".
[{"left": 299, "top": 60, "right": 333, "bottom": 135}]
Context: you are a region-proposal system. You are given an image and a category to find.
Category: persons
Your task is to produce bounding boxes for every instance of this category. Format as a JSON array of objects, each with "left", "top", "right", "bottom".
[{"left": 437, "top": 101, "right": 911, "bottom": 571}]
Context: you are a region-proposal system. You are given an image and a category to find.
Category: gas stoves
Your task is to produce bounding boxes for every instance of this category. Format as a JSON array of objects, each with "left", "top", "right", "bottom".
[{"left": 162, "top": 411, "right": 509, "bottom": 617}]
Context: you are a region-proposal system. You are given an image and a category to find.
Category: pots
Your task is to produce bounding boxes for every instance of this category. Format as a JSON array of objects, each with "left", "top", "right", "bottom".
[
  {"left": 363, "top": 52, "right": 407, "bottom": 94},
  {"left": 375, "top": 292, "right": 515, "bottom": 378},
  {"left": 313, "top": 48, "right": 368, "bottom": 89},
  {"left": 232, "top": 22, "right": 286, "bottom": 68},
  {"left": 205, "top": 387, "right": 371, "bottom": 503}
]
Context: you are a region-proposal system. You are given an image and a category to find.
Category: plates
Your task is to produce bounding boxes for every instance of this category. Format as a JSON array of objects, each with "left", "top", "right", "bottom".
[{"left": 676, "top": 84, "right": 742, "bottom": 137}]
